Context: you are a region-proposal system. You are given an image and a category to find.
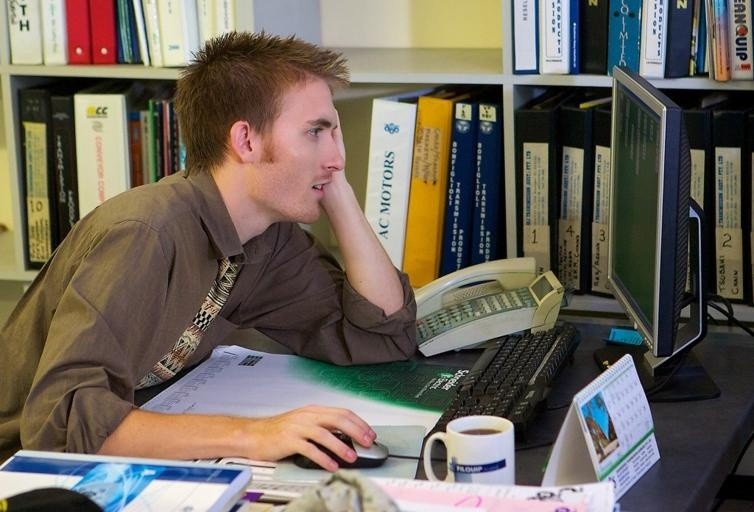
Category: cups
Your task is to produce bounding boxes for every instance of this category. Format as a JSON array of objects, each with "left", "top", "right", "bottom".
[{"left": 424, "top": 413, "right": 516, "bottom": 489}]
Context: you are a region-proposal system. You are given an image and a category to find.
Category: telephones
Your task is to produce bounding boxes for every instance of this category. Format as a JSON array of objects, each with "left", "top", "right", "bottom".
[{"left": 411, "top": 257, "right": 566, "bottom": 358}]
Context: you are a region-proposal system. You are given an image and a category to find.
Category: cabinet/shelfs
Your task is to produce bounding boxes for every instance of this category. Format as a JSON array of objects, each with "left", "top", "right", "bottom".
[{"left": 1, "top": 0, "right": 752, "bottom": 331}]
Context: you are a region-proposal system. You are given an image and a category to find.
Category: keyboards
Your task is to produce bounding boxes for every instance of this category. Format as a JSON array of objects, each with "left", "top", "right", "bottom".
[{"left": 429, "top": 324, "right": 580, "bottom": 444}]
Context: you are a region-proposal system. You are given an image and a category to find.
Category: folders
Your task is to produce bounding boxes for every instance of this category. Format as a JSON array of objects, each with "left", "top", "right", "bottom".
[{"left": 515, "top": 88, "right": 753, "bottom": 307}]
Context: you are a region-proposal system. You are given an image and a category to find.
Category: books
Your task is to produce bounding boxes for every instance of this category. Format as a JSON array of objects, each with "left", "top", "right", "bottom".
[
  {"left": 6, "top": 0, "right": 253, "bottom": 271},
  {"left": 0, "top": 450, "right": 619, "bottom": 512}
]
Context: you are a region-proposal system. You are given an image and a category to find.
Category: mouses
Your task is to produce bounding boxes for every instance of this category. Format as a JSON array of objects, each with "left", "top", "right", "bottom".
[{"left": 292, "top": 430, "right": 391, "bottom": 472}]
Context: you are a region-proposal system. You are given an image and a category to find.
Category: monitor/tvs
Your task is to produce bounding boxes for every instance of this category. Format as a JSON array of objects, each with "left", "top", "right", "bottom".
[{"left": 590, "top": 64, "right": 721, "bottom": 403}]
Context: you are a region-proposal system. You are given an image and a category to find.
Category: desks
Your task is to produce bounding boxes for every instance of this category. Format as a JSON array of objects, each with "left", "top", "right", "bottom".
[{"left": 137, "top": 319, "right": 753, "bottom": 512}]
